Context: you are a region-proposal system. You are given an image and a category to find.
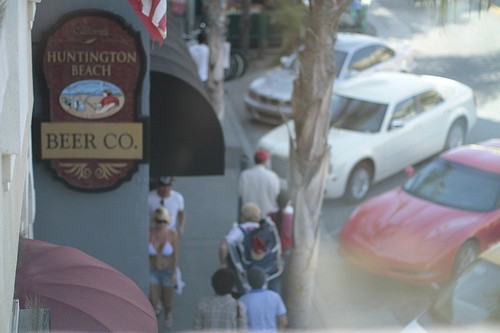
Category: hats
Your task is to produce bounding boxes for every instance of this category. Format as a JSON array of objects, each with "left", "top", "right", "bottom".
[
  {"left": 158, "top": 171, "right": 174, "bottom": 186},
  {"left": 255, "top": 149, "right": 269, "bottom": 160}
]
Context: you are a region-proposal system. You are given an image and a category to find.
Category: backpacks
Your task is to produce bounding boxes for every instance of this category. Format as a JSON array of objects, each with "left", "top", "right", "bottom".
[{"left": 239, "top": 230, "right": 276, "bottom": 278}]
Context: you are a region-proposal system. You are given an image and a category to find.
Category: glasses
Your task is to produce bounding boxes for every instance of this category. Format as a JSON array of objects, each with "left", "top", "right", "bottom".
[{"left": 156, "top": 218, "right": 167, "bottom": 224}]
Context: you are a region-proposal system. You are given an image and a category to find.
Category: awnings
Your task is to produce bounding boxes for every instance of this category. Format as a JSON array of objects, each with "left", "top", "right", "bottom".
[{"left": 140, "top": 12, "right": 224, "bottom": 178}]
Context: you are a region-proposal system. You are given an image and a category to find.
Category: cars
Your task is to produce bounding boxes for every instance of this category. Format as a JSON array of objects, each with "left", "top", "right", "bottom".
[
  {"left": 256, "top": 72, "right": 479, "bottom": 206},
  {"left": 244, "top": 28, "right": 414, "bottom": 129}
]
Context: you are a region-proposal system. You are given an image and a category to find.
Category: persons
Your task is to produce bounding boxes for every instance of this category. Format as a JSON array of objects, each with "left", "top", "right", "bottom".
[
  {"left": 219, "top": 202, "right": 279, "bottom": 292},
  {"left": 147, "top": 208, "right": 180, "bottom": 328},
  {"left": 239, "top": 150, "right": 284, "bottom": 218},
  {"left": 268, "top": 189, "right": 293, "bottom": 260},
  {"left": 147, "top": 175, "right": 189, "bottom": 236},
  {"left": 196, "top": 271, "right": 245, "bottom": 333},
  {"left": 240, "top": 267, "right": 289, "bottom": 333}
]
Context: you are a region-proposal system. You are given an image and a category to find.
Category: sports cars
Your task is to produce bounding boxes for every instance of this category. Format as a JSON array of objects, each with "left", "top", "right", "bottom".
[{"left": 338, "top": 136, "right": 499, "bottom": 302}]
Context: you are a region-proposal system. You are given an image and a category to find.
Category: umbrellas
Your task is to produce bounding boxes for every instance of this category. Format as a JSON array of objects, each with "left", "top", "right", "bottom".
[{"left": 14, "top": 236, "right": 158, "bottom": 333}]
